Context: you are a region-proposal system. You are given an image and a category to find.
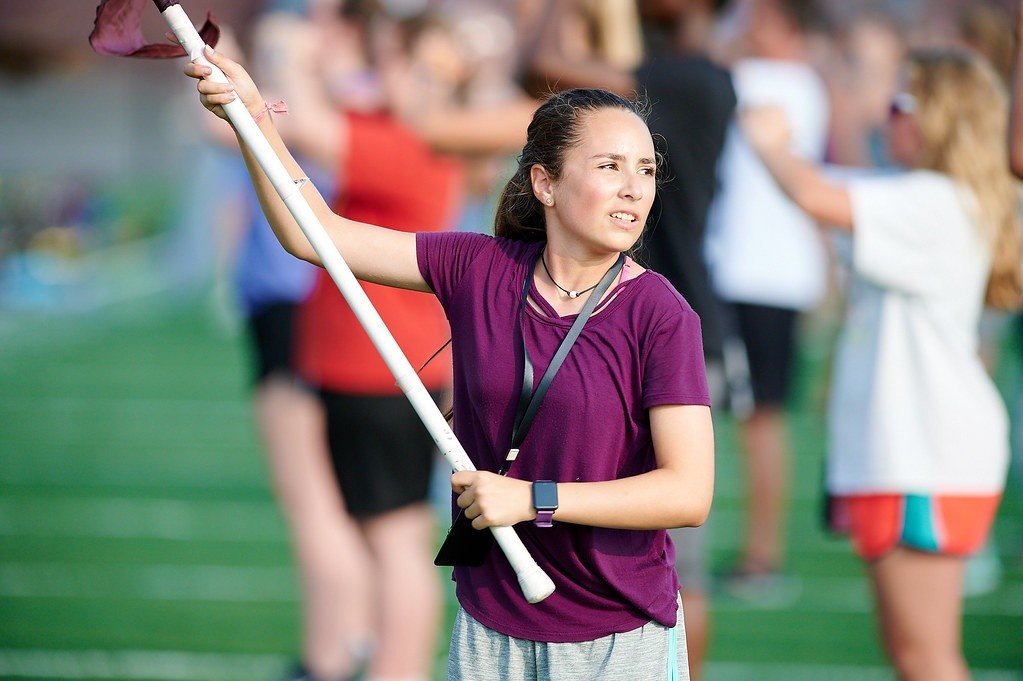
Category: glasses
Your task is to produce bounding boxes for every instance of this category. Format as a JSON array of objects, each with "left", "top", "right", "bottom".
[{"left": 888, "top": 92, "right": 920, "bottom": 118}]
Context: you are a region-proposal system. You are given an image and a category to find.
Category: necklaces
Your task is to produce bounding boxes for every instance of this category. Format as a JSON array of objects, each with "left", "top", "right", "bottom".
[{"left": 541, "top": 245, "right": 622, "bottom": 299}]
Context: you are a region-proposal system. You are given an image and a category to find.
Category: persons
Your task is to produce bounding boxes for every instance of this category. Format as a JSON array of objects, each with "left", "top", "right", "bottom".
[
  {"left": 164, "top": 29, "right": 715, "bottom": 681},
  {"left": 191, "top": 0, "right": 1023, "bottom": 681}
]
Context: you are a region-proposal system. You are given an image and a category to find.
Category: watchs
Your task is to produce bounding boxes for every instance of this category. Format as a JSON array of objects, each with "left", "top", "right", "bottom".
[{"left": 532, "top": 480, "right": 559, "bottom": 528}]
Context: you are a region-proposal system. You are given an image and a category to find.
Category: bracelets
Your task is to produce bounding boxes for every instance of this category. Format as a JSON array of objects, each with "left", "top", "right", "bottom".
[{"left": 231, "top": 101, "right": 288, "bottom": 132}]
